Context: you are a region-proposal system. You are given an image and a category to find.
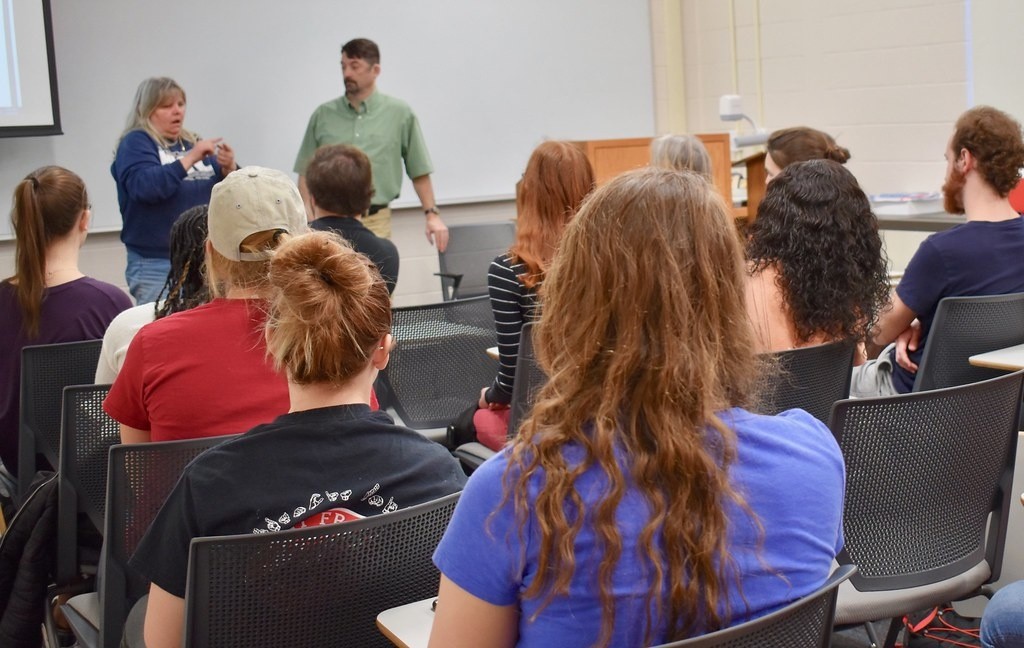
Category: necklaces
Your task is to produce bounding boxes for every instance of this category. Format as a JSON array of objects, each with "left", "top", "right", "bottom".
[{"left": 179, "top": 139, "right": 185, "bottom": 152}]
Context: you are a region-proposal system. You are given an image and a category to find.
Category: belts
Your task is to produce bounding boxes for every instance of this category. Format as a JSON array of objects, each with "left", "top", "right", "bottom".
[{"left": 362, "top": 203, "right": 389, "bottom": 218}]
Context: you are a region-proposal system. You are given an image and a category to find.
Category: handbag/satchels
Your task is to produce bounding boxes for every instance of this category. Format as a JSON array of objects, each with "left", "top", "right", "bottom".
[{"left": 895, "top": 601, "right": 982, "bottom": 648}]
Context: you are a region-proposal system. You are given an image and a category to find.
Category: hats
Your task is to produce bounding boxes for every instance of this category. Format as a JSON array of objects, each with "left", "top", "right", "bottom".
[{"left": 207, "top": 165, "right": 308, "bottom": 262}]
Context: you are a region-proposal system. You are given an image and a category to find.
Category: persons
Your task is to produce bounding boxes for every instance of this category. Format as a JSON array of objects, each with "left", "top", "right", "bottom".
[
  {"left": 305, "top": 145, "right": 400, "bottom": 298},
  {"left": 849, "top": 106, "right": 1024, "bottom": 399},
  {"left": 82, "top": 206, "right": 209, "bottom": 440},
  {"left": 765, "top": 127, "right": 850, "bottom": 185},
  {"left": 102, "top": 165, "right": 378, "bottom": 560},
  {"left": 745, "top": 159, "right": 890, "bottom": 365},
  {"left": 119, "top": 233, "right": 472, "bottom": 648},
  {"left": 0, "top": 166, "right": 135, "bottom": 482},
  {"left": 111, "top": 77, "right": 240, "bottom": 304},
  {"left": 980, "top": 580, "right": 1024, "bottom": 648},
  {"left": 429, "top": 168, "right": 846, "bottom": 648},
  {"left": 293, "top": 39, "right": 448, "bottom": 253},
  {"left": 452, "top": 140, "right": 595, "bottom": 448},
  {"left": 651, "top": 136, "right": 710, "bottom": 176}
]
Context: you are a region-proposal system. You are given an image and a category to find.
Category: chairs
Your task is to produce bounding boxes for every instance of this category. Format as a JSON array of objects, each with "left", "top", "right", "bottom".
[{"left": 0, "top": 223, "right": 1024, "bottom": 648}]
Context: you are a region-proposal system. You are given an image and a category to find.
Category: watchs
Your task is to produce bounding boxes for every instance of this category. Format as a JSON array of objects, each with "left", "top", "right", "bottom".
[{"left": 425, "top": 207, "right": 440, "bottom": 215}]
